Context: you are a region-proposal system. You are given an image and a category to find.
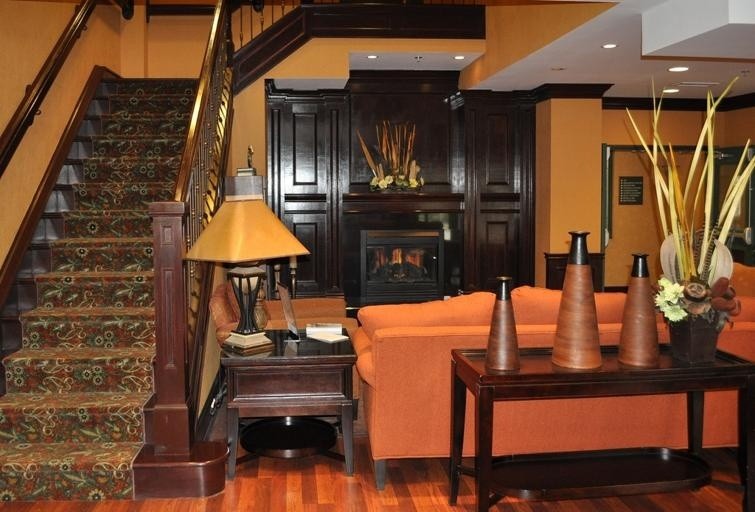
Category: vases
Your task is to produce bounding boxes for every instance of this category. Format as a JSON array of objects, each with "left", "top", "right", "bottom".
[{"left": 665, "top": 314, "right": 721, "bottom": 363}]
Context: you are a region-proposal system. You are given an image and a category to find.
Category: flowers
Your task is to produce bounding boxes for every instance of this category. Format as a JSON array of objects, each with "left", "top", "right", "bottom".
[
  {"left": 354, "top": 120, "right": 426, "bottom": 192},
  {"left": 624, "top": 72, "right": 755, "bottom": 365}
]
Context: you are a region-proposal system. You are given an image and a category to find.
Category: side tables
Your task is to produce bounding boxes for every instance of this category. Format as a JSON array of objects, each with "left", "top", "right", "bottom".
[{"left": 220, "top": 326, "right": 362, "bottom": 478}]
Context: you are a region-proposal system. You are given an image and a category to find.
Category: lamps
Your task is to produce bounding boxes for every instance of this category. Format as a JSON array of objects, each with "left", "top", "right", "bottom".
[{"left": 182, "top": 167, "right": 312, "bottom": 356}]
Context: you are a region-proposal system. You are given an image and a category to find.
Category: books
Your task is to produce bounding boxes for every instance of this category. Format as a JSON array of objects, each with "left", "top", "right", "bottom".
[
  {"left": 306, "top": 323, "right": 342, "bottom": 335},
  {"left": 308, "top": 333, "right": 348, "bottom": 344}
]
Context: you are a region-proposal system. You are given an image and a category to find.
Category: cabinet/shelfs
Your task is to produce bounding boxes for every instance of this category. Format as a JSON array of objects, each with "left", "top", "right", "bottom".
[{"left": 263, "top": 70, "right": 536, "bottom": 323}]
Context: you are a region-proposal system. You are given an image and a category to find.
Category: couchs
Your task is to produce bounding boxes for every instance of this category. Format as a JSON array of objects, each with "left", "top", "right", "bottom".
[
  {"left": 208, "top": 280, "right": 359, "bottom": 341},
  {"left": 348, "top": 261, "right": 755, "bottom": 491}
]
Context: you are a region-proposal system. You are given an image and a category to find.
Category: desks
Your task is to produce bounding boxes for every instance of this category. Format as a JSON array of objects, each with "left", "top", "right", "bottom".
[{"left": 448, "top": 345, "right": 753, "bottom": 511}]
